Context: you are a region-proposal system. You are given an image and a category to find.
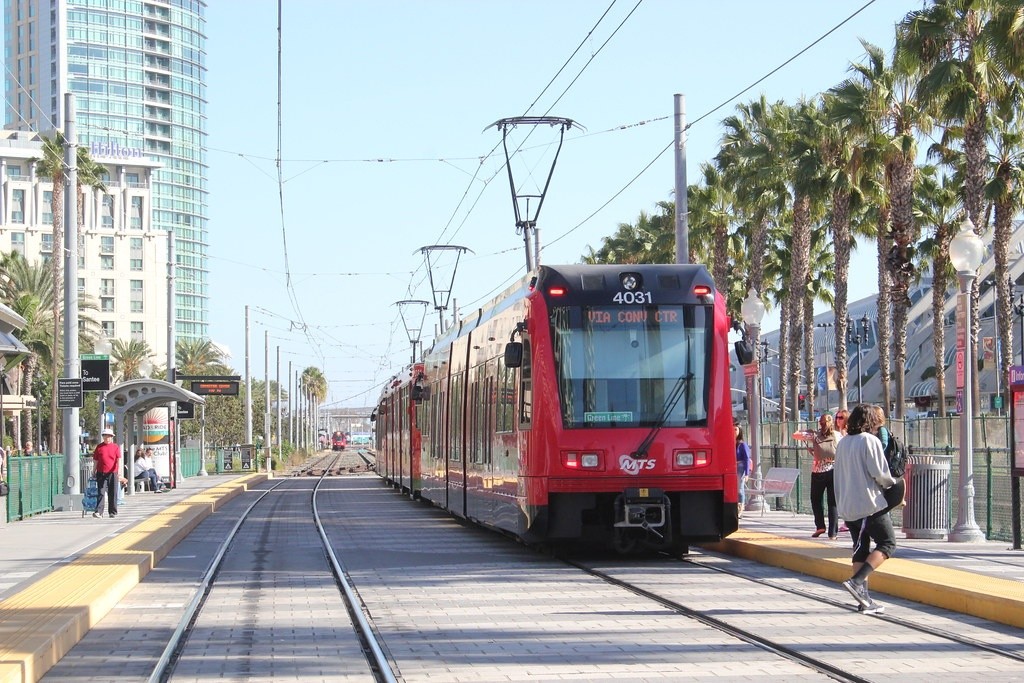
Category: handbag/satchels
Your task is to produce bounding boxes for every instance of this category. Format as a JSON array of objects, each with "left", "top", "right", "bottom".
[{"left": 0, "top": 481, "right": 8, "bottom": 496}]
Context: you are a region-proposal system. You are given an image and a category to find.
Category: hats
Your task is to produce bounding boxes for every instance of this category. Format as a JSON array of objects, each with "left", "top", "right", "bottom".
[{"left": 101, "top": 429, "right": 116, "bottom": 436}]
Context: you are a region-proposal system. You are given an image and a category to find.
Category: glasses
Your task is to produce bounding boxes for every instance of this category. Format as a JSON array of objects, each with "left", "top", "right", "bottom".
[{"left": 836, "top": 416, "right": 844, "bottom": 420}]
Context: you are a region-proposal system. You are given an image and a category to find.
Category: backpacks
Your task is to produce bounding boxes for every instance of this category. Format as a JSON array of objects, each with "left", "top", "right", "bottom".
[
  {"left": 738, "top": 440, "right": 753, "bottom": 471},
  {"left": 815, "top": 435, "right": 837, "bottom": 458},
  {"left": 878, "top": 426, "right": 906, "bottom": 477}
]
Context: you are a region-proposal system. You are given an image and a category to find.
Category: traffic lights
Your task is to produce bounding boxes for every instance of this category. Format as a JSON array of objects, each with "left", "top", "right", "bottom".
[{"left": 798, "top": 394, "right": 805, "bottom": 411}]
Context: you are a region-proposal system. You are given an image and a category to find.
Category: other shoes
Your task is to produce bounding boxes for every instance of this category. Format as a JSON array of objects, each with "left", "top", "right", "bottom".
[
  {"left": 738, "top": 513, "right": 743, "bottom": 519},
  {"left": 838, "top": 527, "right": 850, "bottom": 532},
  {"left": 109, "top": 513, "right": 115, "bottom": 518},
  {"left": 812, "top": 529, "right": 825, "bottom": 537},
  {"left": 155, "top": 488, "right": 170, "bottom": 493},
  {"left": 829, "top": 537, "right": 837, "bottom": 540},
  {"left": 93, "top": 513, "right": 103, "bottom": 518}
]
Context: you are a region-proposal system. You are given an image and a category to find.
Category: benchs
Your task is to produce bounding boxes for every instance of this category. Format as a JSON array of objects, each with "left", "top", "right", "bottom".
[
  {"left": 124, "top": 464, "right": 152, "bottom": 493},
  {"left": 743, "top": 467, "right": 800, "bottom": 518}
]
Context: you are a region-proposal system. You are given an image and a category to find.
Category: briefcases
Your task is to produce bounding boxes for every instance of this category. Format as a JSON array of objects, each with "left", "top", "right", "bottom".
[{"left": 82, "top": 478, "right": 99, "bottom": 518}]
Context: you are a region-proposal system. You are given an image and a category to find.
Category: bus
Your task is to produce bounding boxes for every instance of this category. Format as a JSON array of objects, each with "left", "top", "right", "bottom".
[
  {"left": 331, "top": 431, "right": 347, "bottom": 452},
  {"left": 375, "top": 261, "right": 755, "bottom": 555}
]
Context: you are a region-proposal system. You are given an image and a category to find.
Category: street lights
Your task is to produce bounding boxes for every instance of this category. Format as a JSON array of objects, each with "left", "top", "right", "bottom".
[
  {"left": 984, "top": 280, "right": 1001, "bottom": 418},
  {"left": 847, "top": 312, "right": 871, "bottom": 407},
  {"left": 1009, "top": 279, "right": 1024, "bottom": 365},
  {"left": 760, "top": 339, "right": 770, "bottom": 417},
  {"left": 817, "top": 322, "right": 835, "bottom": 412},
  {"left": 950, "top": 208, "right": 988, "bottom": 540}
]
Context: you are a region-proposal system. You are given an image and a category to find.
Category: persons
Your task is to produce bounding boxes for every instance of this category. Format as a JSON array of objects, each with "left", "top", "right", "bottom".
[
  {"left": 0, "top": 441, "right": 36, "bottom": 486},
  {"left": 833, "top": 403, "right": 896, "bottom": 616},
  {"left": 121, "top": 448, "right": 170, "bottom": 494},
  {"left": 735, "top": 426, "right": 753, "bottom": 518},
  {"left": 792, "top": 406, "right": 889, "bottom": 540},
  {"left": 92, "top": 429, "right": 122, "bottom": 518}
]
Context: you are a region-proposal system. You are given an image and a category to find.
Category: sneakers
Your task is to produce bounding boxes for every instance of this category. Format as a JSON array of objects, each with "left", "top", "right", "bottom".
[
  {"left": 842, "top": 578, "right": 872, "bottom": 607},
  {"left": 858, "top": 600, "right": 883, "bottom": 614}
]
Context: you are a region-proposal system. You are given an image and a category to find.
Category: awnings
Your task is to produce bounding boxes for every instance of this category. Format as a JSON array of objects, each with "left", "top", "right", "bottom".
[{"left": 908, "top": 382, "right": 938, "bottom": 398}]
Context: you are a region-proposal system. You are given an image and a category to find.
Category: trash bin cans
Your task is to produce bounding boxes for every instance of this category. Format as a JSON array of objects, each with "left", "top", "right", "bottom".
[{"left": 903, "top": 455, "right": 954, "bottom": 538}]
